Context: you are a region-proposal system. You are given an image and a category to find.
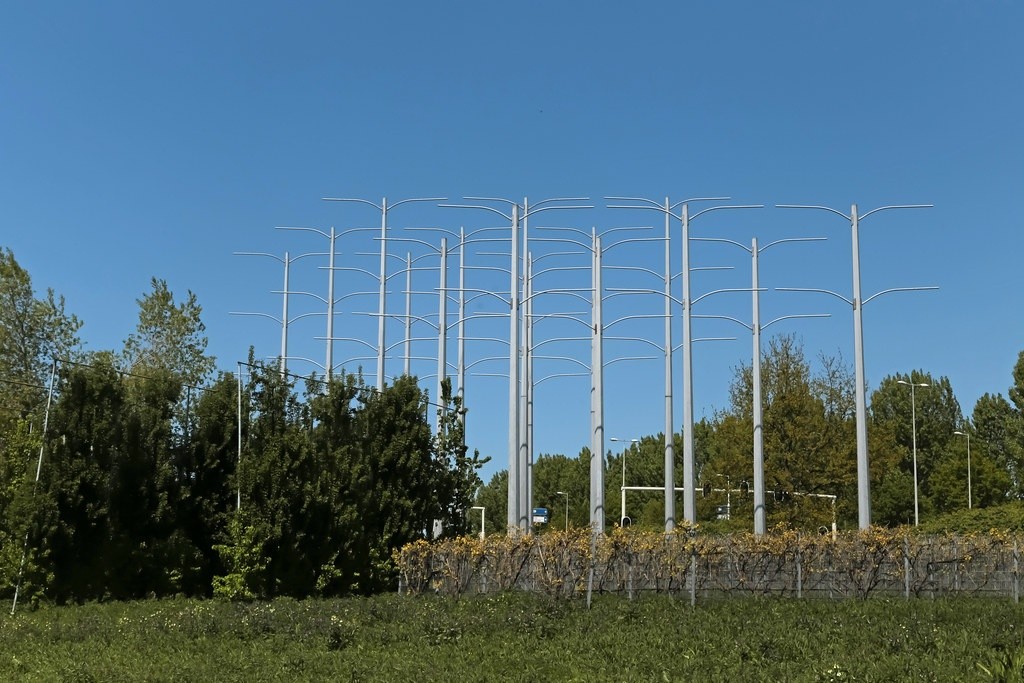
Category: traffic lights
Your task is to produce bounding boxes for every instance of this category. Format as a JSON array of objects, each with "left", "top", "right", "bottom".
[
  {"left": 774, "top": 482, "right": 783, "bottom": 504},
  {"left": 704, "top": 481, "right": 713, "bottom": 500},
  {"left": 622, "top": 517, "right": 632, "bottom": 529},
  {"left": 740, "top": 480, "right": 750, "bottom": 504}
]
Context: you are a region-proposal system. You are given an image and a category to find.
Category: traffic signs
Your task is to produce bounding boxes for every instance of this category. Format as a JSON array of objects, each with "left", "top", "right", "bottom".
[
  {"left": 532, "top": 508, "right": 548, "bottom": 515},
  {"left": 533, "top": 515, "right": 548, "bottom": 523}
]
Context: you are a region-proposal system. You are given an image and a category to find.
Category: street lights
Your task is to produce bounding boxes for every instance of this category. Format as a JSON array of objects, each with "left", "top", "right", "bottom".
[
  {"left": 953, "top": 430, "right": 971, "bottom": 510},
  {"left": 897, "top": 380, "right": 930, "bottom": 526},
  {"left": 609, "top": 438, "right": 639, "bottom": 527},
  {"left": 471, "top": 506, "right": 486, "bottom": 541},
  {"left": 717, "top": 473, "right": 731, "bottom": 522},
  {"left": 556, "top": 492, "right": 569, "bottom": 533}
]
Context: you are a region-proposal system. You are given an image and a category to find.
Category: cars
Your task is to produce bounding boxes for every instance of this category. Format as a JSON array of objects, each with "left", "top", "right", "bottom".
[{"left": 715, "top": 504, "right": 733, "bottom": 520}]
